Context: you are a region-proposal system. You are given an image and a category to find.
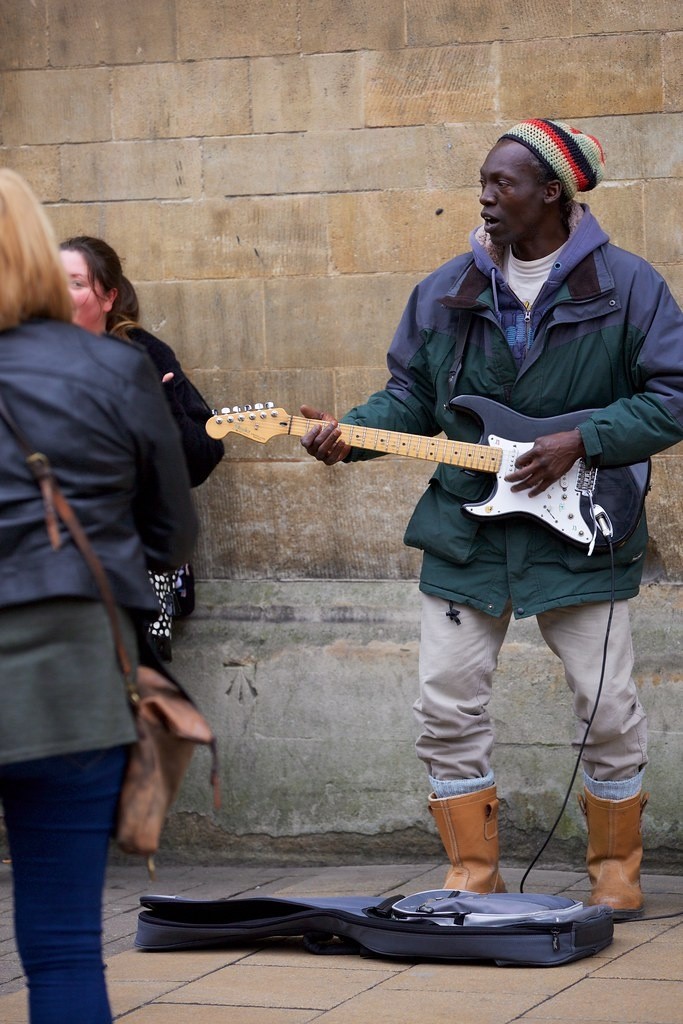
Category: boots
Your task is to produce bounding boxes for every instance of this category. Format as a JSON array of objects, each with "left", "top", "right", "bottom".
[
  {"left": 576, "top": 782, "right": 649, "bottom": 919},
  {"left": 426, "top": 785, "right": 508, "bottom": 895}
]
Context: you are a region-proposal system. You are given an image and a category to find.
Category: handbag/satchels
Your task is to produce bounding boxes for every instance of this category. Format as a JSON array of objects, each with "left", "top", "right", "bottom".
[{"left": 113, "top": 665, "right": 223, "bottom": 879}]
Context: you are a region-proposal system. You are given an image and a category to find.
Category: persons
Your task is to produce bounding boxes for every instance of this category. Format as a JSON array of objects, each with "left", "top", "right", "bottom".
[
  {"left": 0, "top": 170, "right": 202, "bottom": 1024},
  {"left": 54, "top": 237, "right": 227, "bottom": 662},
  {"left": 301, "top": 117, "right": 683, "bottom": 920}
]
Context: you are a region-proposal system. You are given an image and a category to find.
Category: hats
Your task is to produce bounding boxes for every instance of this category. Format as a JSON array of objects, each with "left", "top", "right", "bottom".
[{"left": 494, "top": 118, "right": 604, "bottom": 200}]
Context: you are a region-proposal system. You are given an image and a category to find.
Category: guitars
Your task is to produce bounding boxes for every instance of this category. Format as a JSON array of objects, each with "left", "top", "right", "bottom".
[{"left": 205, "top": 394, "right": 652, "bottom": 555}]
